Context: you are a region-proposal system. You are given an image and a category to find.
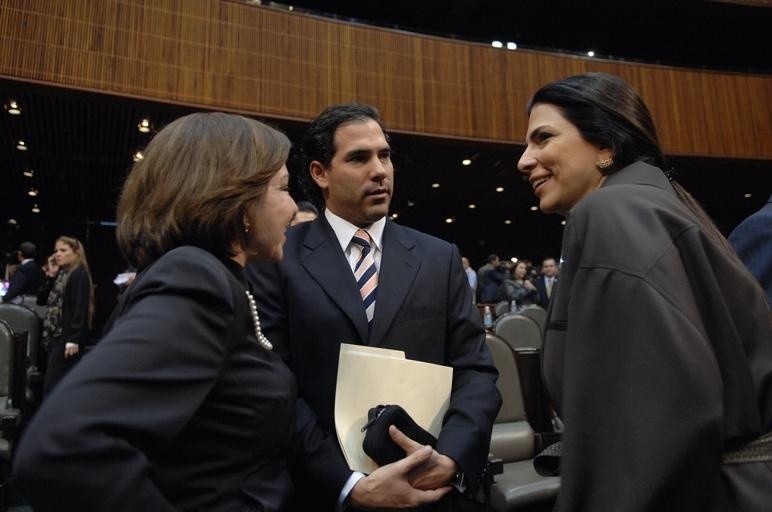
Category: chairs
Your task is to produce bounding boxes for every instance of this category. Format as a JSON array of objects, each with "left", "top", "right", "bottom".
[
  {"left": 477, "top": 330, "right": 564, "bottom": 512},
  {"left": 0, "top": 293, "right": 59, "bottom": 466},
  {"left": 491, "top": 303, "right": 554, "bottom": 356}
]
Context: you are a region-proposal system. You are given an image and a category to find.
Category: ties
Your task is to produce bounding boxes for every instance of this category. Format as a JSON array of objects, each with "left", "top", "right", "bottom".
[{"left": 352, "top": 231, "right": 379, "bottom": 327}]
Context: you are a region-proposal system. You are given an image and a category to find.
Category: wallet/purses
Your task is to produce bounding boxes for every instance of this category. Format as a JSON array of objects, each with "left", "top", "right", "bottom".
[{"left": 361, "top": 403, "right": 438, "bottom": 467}]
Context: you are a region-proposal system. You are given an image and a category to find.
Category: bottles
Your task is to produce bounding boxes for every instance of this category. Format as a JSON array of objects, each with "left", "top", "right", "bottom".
[
  {"left": 510, "top": 300, "right": 518, "bottom": 315},
  {"left": 483, "top": 305, "right": 493, "bottom": 330}
]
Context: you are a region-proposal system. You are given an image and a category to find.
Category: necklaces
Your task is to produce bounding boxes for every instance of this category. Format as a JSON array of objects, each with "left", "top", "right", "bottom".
[{"left": 244, "top": 290, "right": 272, "bottom": 351}]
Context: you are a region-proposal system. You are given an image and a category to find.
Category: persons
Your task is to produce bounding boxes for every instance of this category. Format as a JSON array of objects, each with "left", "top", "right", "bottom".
[
  {"left": 11, "top": 111, "right": 307, "bottom": 512},
  {"left": 461, "top": 256, "right": 562, "bottom": 327},
  {"left": 250, "top": 104, "right": 504, "bottom": 509},
  {"left": 291, "top": 200, "right": 321, "bottom": 224},
  {"left": 1, "top": 236, "right": 93, "bottom": 393},
  {"left": 729, "top": 197, "right": 771, "bottom": 290},
  {"left": 517, "top": 74, "right": 771, "bottom": 511}
]
